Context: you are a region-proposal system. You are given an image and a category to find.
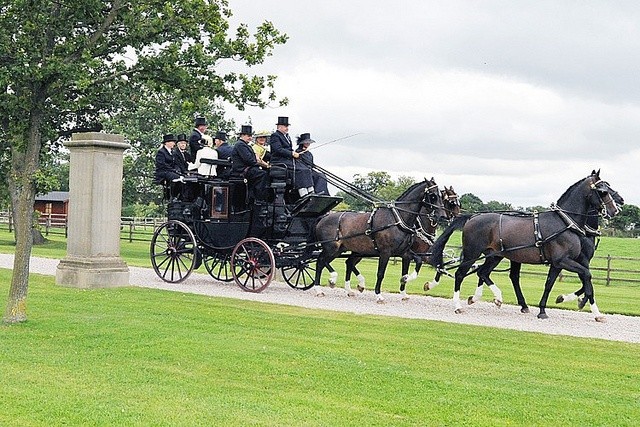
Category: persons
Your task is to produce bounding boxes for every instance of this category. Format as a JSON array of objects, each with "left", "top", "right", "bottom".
[
  {"left": 270, "top": 116, "right": 315, "bottom": 198},
  {"left": 214, "top": 131, "right": 235, "bottom": 177},
  {"left": 186, "top": 135, "right": 218, "bottom": 177},
  {"left": 231, "top": 125, "right": 267, "bottom": 196},
  {"left": 156, "top": 134, "right": 183, "bottom": 184},
  {"left": 295, "top": 133, "right": 330, "bottom": 195},
  {"left": 189, "top": 117, "right": 207, "bottom": 164},
  {"left": 252, "top": 130, "right": 271, "bottom": 169},
  {"left": 174, "top": 133, "right": 191, "bottom": 173}
]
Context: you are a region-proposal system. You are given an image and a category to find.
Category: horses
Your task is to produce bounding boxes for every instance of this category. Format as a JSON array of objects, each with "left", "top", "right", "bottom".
[
  {"left": 467, "top": 183, "right": 625, "bottom": 320},
  {"left": 303, "top": 176, "right": 451, "bottom": 304},
  {"left": 428, "top": 168, "right": 620, "bottom": 323},
  {"left": 344, "top": 184, "right": 462, "bottom": 297}
]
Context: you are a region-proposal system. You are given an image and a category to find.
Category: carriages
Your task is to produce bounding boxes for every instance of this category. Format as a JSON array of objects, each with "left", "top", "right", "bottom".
[{"left": 149, "top": 167, "right": 625, "bottom": 325}]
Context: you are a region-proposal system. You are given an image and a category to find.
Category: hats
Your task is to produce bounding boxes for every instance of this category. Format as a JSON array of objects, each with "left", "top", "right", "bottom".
[
  {"left": 297, "top": 133, "right": 315, "bottom": 145},
  {"left": 196, "top": 118, "right": 208, "bottom": 126},
  {"left": 238, "top": 126, "right": 254, "bottom": 135},
  {"left": 276, "top": 117, "right": 290, "bottom": 125},
  {"left": 176, "top": 134, "right": 188, "bottom": 143},
  {"left": 214, "top": 132, "right": 227, "bottom": 140},
  {"left": 254, "top": 131, "right": 273, "bottom": 137},
  {"left": 198, "top": 135, "right": 213, "bottom": 147},
  {"left": 161, "top": 134, "right": 176, "bottom": 143}
]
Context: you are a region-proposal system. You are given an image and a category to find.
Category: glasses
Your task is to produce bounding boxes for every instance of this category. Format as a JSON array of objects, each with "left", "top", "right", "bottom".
[{"left": 258, "top": 137, "right": 266, "bottom": 138}]
